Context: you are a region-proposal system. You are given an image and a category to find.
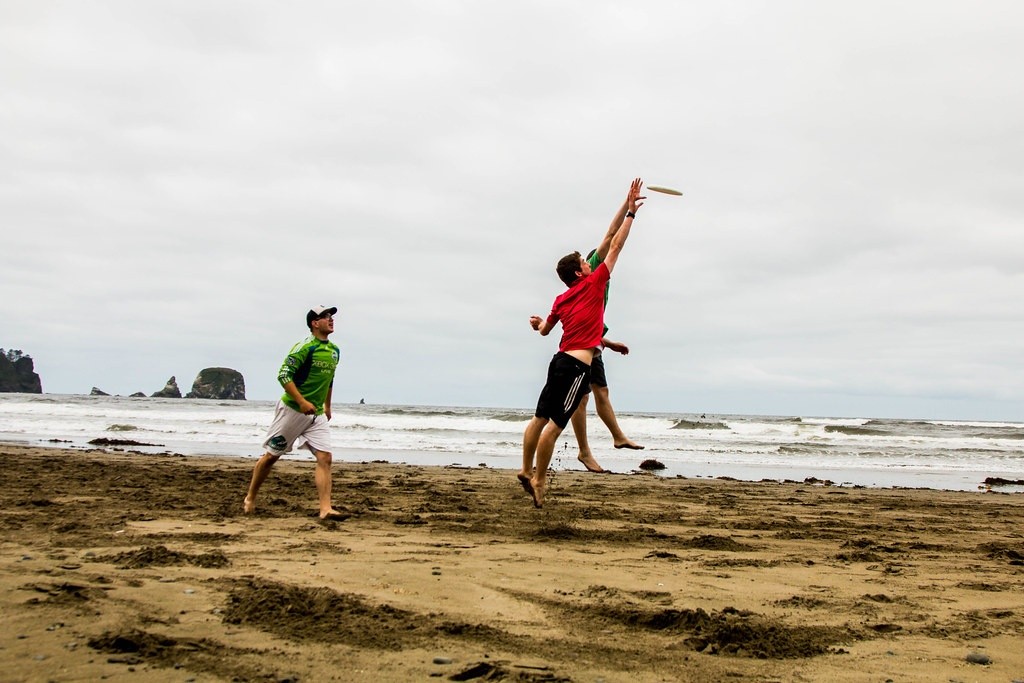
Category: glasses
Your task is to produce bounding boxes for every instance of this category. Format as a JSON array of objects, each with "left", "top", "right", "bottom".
[{"left": 316, "top": 314, "right": 334, "bottom": 321}]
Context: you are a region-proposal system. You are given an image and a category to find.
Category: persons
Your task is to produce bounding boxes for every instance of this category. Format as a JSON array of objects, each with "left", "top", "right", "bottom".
[
  {"left": 244, "top": 303, "right": 343, "bottom": 519},
  {"left": 517, "top": 189, "right": 644, "bottom": 508},
  {"left": 570, "top": 177, "right": 647, "bottom": 473}
]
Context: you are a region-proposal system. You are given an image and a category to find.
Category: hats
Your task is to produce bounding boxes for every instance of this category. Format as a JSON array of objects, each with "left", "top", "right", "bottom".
[{"left": 306, "top": 305, "right": 337, "bottom": 328}]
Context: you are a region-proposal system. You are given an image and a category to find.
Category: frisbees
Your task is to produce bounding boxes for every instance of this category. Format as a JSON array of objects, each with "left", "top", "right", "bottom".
[{"left": 647, "top": 186, "right": 682, "bottom": 197}]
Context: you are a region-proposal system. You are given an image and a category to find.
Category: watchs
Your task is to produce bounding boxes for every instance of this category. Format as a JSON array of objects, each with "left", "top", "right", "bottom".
[{"left": 625, "top": 210, "right": 635, "bottom": 219}]
